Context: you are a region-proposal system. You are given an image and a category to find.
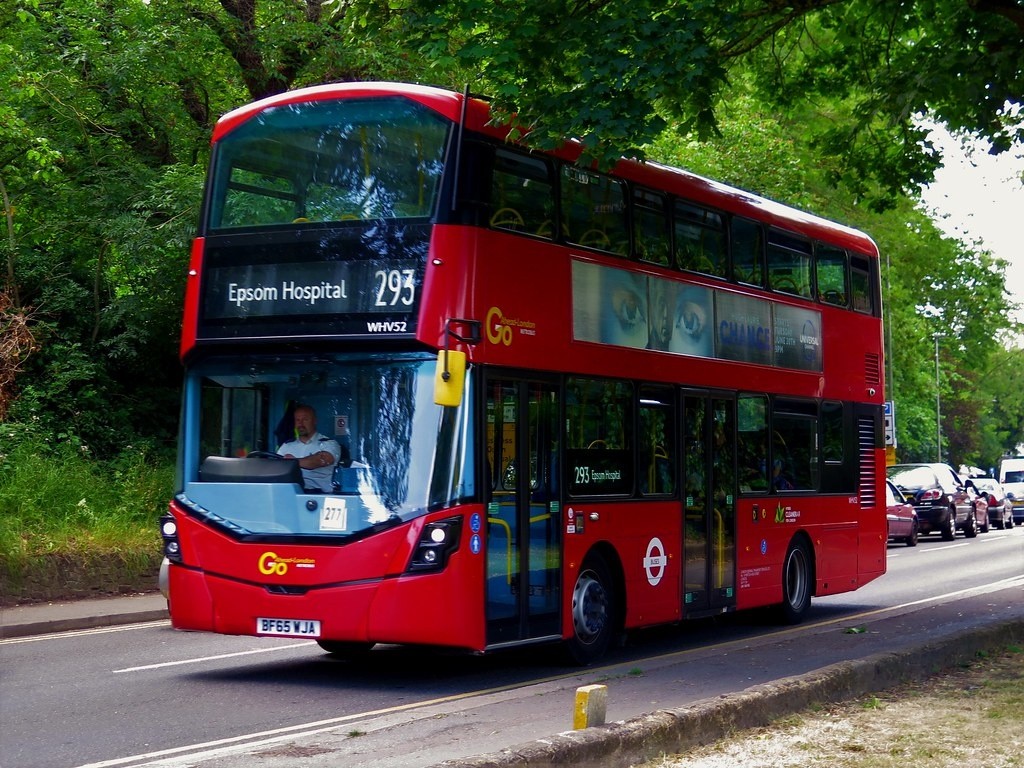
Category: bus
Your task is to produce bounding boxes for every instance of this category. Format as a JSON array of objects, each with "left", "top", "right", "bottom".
[{"left": 158, "top": 79, "right": 888, "bottom": 678}]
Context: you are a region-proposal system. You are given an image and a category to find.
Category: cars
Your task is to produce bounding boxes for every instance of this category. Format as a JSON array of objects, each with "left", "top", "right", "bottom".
[
  {"left": 962, "top": 478, "right": 1015, "bottom": 530},
  {"left": 885, "top": 477, "right": 920, "bottom": 546}
]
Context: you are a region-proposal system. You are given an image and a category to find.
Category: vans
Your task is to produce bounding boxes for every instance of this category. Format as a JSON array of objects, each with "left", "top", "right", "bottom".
[{"left": 996, "top": 458, "right": 1024, "bottom": 525}]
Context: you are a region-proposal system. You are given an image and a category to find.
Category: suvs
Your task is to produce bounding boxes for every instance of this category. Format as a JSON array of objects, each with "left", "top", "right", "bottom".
[{"left": 885, "top": 462, "right": 978, "bottom": 541}]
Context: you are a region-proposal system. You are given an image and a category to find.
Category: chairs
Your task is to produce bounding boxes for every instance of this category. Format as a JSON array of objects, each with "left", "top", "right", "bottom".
[
  {"left": 584, "top": 441, "right": 669, "bottom": 461},
  {"left": 490, "top": 207, "right": 847, "bottom": 306}
]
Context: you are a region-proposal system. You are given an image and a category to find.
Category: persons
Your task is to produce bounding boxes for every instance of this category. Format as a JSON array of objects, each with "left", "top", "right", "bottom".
[
  {"left": 275, "top": 405, "right": 342, "bottom": 493},
  {"left": 659, "top": 425, "right": 794, "bottom": 501}
]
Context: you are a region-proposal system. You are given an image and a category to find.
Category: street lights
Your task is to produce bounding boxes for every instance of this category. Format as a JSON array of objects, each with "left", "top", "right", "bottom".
[{"left": 932, "top": 331, "right": 946, "bottom": 463}]
